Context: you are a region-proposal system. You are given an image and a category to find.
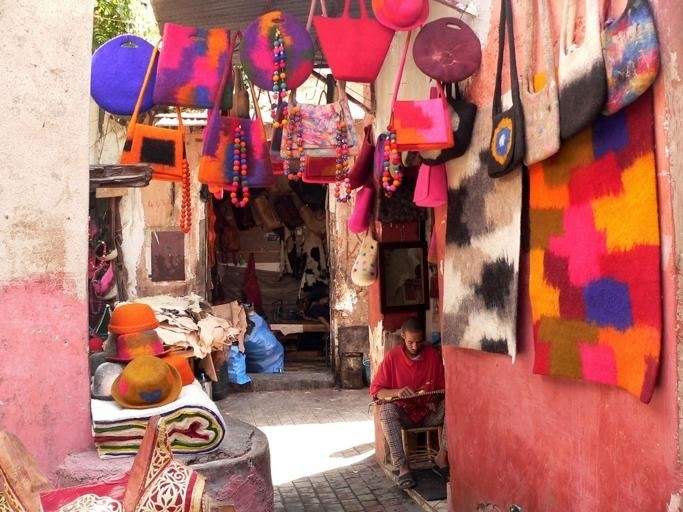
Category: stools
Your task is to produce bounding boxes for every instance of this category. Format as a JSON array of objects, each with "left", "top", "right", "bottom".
[{"left": 382, "top": 426, "right": 443, "bottom": 471}]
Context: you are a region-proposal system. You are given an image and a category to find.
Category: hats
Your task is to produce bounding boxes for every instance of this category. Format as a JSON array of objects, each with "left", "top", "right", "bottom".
[
  {"left": 108, "top": 303, "right": 161, "bottom": 335},
  {"left": 90, "top": 362, "right": 125, "bottom": 401},
  {"left": 103, "top": 330, "right": 172, "bottom": 363},
  {"left": 161, "top": 353, "right": 195, "bottom": 386},
  {"left": 110, "top": 355, "right": 183, "bottom": 410},
  {"left": 372, "top": 1, "right": 431, "bottom": 31}
]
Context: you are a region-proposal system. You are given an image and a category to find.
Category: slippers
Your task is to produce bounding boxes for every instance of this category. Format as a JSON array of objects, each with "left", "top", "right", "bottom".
[
  {"left": 433, "top": 462, "right": 450, "bottom": 481},
  {"left": 397, "top": 469, "right": 416, "bottom": 490}
]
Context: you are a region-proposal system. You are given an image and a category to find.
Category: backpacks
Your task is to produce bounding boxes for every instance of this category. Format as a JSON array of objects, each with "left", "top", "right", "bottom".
[{"left": 310, "top": 15, "right": 395, "bottom": 84}]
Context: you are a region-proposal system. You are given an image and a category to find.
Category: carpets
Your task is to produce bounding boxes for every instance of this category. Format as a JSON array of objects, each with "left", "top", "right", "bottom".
[
  {"left": 514, "top": 85, "right": 664, "bottom": 405},
  {"left": 443, "top": 0, "right": 526, "bottom": 364}
]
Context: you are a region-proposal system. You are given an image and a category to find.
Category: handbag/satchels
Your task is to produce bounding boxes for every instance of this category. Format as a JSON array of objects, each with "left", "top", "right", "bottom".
[
  {"left": 280, "top": 98, "right": 363, "bottom": 159},
  {"left": 220, "top": 224, "right": 240, "bottom": 253},
  {"left": 412, "top": 162, "right": 450, "bottom": 209},
  {"left": 226, "top": 90, "right": 250, "bottom": 120},
  {"left": 213, "top": 209, "right": 230, "bottom": 234},
  {"left": 373, "top": 134, "right": 388, "bottom": 183},
  {"left": 92, "top": 262, "right": 117, "bottom": 301},
  {"left": 487, "top": 101, "right": 526, "bottom": 178},
  {"left": 348, "top": 124, "right": 374, "bottom": 190},
  {"left": 348, "top": 187, "right": 374, "bottom": 234},
  {"left": 302, "top": 156, "right": 346, "bottom": 185},
  {"left": 287, "top": 171, "right": 327, "bottom": 212},
  {"left": 273, "top": 193, "right": 304, "bottom": 230},
  {"left": 197, "top": 114, "right": 275, "bottom": 188},
  {"left": 153, "top": 21, "right": 233, "bottom": 110},
  {"left": 555, "top": 36, "right": 609, "bottom": 142},
  {"left": 349, "top": 225, "right": 380, "bottom": 286},
  {"left": 414, "top": 97, "right": 478, "bottom": 166},
  {"left": 119, "top": 123, "right": 186, "bottom": 182},
  {"left": 254, "top": 194, "right": 282, "bottom": 230},
  {"left": 228, "top": 195, "right": 255, "bottom": 232},
  {"left": 90, "top": 34, "right": 160, "bottom": 117},
  {"left": 392, "top": 97, "right": 455, "bottom": 152},
  {"left": 412, "top": 17, "right": 482, "bottom": 83},
  {"left": 596, "top": 1, "right": 661, "bottom": 116},
  {"left": 297, "top": 204, "right": 326, "bottom": 237},
  {"left": 239, "top": 10, "right": 315, "bottom": 93},
  {"left": 520, "top": 77, "right": 560, "bottom": 167}
]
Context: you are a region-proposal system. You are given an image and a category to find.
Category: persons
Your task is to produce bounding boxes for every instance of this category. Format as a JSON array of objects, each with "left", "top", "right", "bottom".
[
  {"left": 307, "top": 282, "right": 330, "bottom": 330},
  {"left": 369, "top": 320, "right": 449, "bottom": 487}
]
